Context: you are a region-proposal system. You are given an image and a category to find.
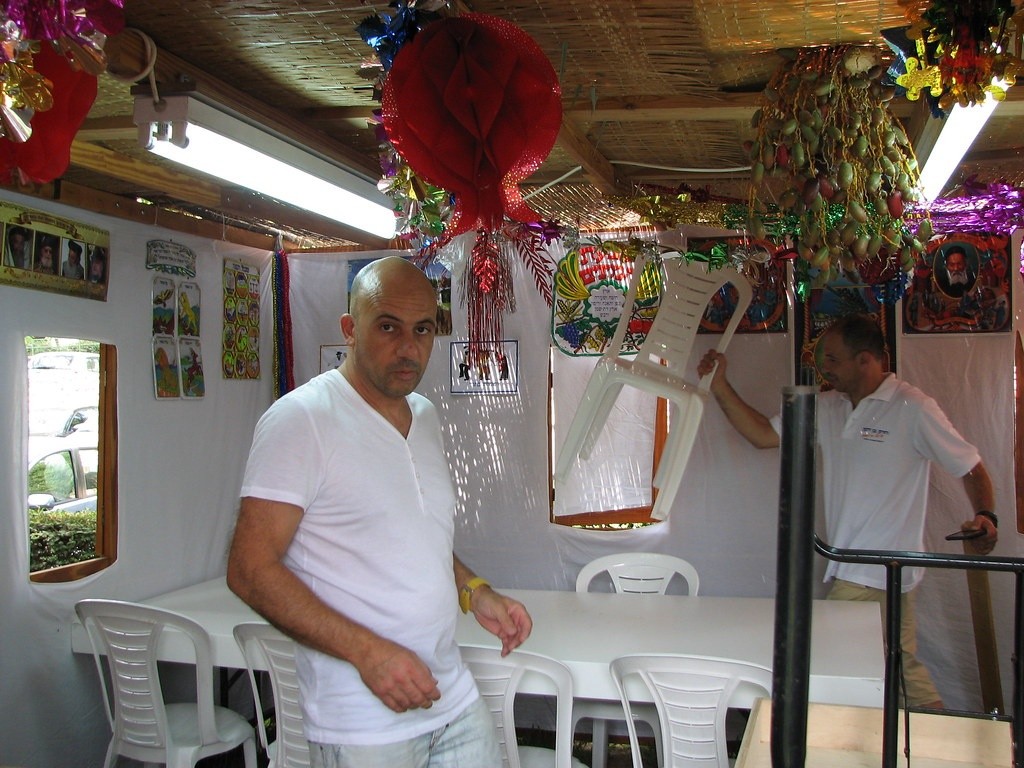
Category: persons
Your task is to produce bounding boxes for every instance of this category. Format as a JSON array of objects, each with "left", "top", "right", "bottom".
[
  {"left": 697, "top": 310, "right": 999, "bottom": 709},
  {"left": 35, "top": 232, "right": 56, "bottom": 275},
  {"left": 327, "top": 351, "right": 347, "bottom": 369},
  {"left": 4, "top": 227, "right": 33, "bottom": 270},
  {"left": 939, "top": 245, "right": 977, "bottom": 298},
  {"left": 61, "top": 239, "right": 84, "bottom": 280},
  {"left": 89, "top": 245, "right": 105, "bottom": 283},
  {"left": 226, "top": 255, "right": 533, "bottom": 768}
]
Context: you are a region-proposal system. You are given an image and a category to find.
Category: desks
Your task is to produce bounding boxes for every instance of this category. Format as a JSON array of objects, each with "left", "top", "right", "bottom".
[{"left": 77, "top": 575, "right": 884, "bottom": 768}]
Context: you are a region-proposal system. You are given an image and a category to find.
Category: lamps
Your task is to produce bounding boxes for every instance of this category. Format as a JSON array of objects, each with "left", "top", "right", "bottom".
[
  {"left": 127, "top": 86, "right": 416, "bottom": 244},
  {"left": 901, "top": 40, "right": 1016, "bottom": 201}
]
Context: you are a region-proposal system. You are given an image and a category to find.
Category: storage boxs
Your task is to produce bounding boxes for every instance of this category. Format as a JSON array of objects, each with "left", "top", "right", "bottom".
[{"left": 735, "top": 696, "right": 1014, "bottom": 768}]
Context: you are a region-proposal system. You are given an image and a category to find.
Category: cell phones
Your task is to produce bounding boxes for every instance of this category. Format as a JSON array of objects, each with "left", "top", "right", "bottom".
[{"left": 945, "top": 530, "right": 984, "bottom": 540}]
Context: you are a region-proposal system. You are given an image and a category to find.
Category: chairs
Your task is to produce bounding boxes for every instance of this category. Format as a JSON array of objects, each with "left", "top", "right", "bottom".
[
  {"left": 73, "top": 597, "right": 262, "bottom": 768},
  {"left": 551, "top": 250, "right": 755, "bottom": 520},
  {"left": 230, "top": 621, "right": 318, "bottom": 768},
  {"left": 457, "top": 642, "right": 590, "bottom": 768},
  {"left": 572, "top": 553, "right": 701, "bottom": 599},
  {"left": 610, "top": 651, "right": 773, "bottom": 768}
]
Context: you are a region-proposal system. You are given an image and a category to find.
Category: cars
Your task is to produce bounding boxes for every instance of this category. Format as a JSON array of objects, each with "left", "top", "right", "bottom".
[
  {"left": 27, "top": 351, "right": 99, "bottom": 436},
  {"left": 28, "top": 434, "right": 97, "bottom": 516}
]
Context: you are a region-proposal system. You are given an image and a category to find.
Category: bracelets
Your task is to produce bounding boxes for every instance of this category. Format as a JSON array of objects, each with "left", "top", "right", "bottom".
[
  {"left": 459, "top": 577, "right": 493, "bottom": 615},
  {"left": 976, "top": 511, "right": 998, "bottom": 528}
]
